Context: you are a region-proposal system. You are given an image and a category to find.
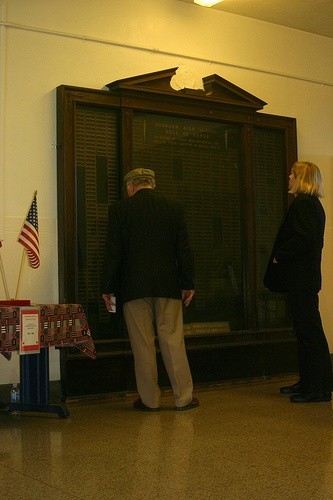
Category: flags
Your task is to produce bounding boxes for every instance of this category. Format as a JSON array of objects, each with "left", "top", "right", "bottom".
[{"left": 17, "top": 196, "right": 41, "bottom": 269}]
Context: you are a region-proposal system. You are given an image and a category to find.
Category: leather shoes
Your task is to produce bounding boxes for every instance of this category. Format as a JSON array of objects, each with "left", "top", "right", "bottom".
[{"left": 280, "top": 382, "right": 332, "bottom": 402}]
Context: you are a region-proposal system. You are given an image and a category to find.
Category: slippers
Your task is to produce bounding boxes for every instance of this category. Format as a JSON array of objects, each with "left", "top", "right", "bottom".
[
  {"left": 176, "top": 397, "right": 199, "bottom": 411},
  {"left": 133, "top": 397, "right": 161, "bottom": 411}
]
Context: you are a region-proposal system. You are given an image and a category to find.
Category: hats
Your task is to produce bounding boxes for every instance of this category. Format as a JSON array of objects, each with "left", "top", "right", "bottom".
[{"left": 123, "top": 168, "right": 155, "bottom": 181}]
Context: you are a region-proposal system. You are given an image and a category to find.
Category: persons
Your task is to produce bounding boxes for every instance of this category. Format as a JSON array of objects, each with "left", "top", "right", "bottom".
[
  {"left": 100, "top": 168, "right": 200, "bottom": 411},
  {"left": 263, "top": 162, "right": 333, "bottom": 402}
]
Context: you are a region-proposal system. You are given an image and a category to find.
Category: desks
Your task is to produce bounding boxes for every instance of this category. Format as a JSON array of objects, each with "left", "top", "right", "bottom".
[{"left": 0, "top": 304, "right": 97, "bottom": 420}]
